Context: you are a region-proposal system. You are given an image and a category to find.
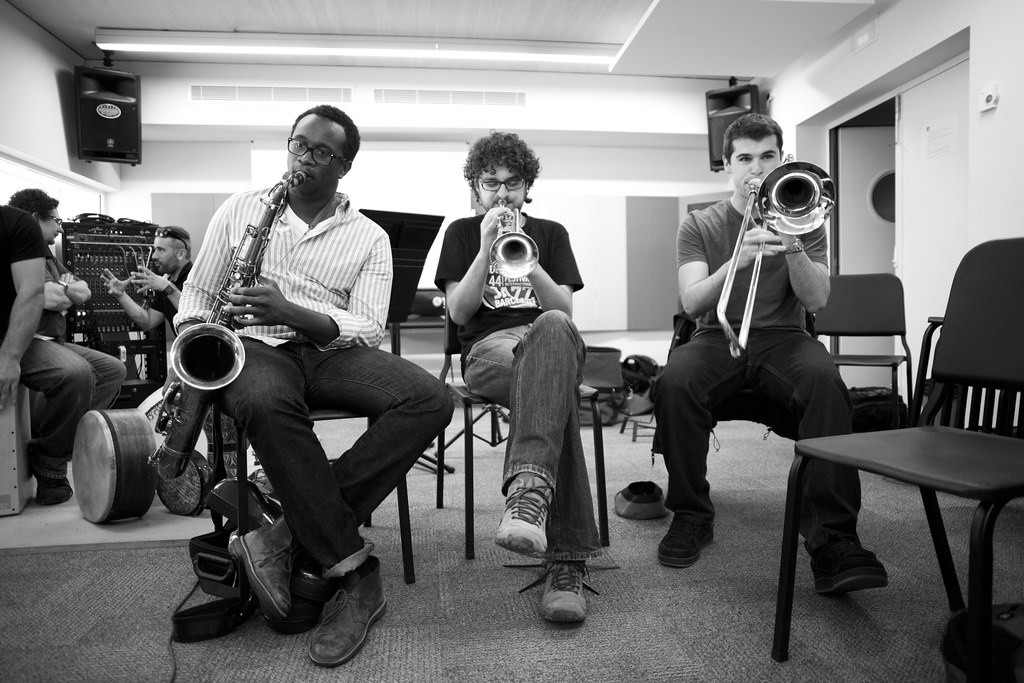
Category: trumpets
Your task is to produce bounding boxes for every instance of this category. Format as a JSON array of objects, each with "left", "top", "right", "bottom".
[{"left": 489, "top": 200, "right": 540, "bottom": 279}]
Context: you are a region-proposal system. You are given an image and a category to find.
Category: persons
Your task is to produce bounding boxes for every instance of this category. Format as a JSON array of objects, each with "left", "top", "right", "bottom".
[
  {"left": 650, "top": 113, "right": 888, "bottom": 594},
  {"left": 0, "top": 188, "right": 127, "bottom": 505},
  {"left": 434, "top": 132, "right": 603, "bottom": 623},
  {"left": 100, "top": 225, "right": 194, "bottom": 338},
  {"left": 173, "top": 105, "right": 456, "bottom": 668}
]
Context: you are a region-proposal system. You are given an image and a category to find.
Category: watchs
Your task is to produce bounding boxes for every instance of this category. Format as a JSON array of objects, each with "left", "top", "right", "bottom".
[
  {"left": 783, "top": 238, "right": 804, "bottom": 255},
  {"left": 162, "top": 282, "right": 176, "bottom": 297}
]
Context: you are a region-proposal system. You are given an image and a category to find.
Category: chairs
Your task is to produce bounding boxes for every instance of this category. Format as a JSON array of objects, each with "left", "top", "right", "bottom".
[
  {"left": 769, "top": 236, "right": 1024, "bottom": 661},
  {"left": 208, "top": 296, "right": 609, "bottom": 587},
  {"left": 814, "top": 273, "right": 913, "bottom": 430}
]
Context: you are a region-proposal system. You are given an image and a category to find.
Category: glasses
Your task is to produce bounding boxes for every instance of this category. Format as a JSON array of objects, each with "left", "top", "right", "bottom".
[
  {"left": 155, "top": 229, "right": 188, "bottom": 249},
  {"left": 287, "top": 136, "right": 346, "bottom": 167},
  {"left": 48, "top": 214, "right": 62, "bottom": 225},
  {"left": 478, "top": 174, "right": 525, "bottom": 192}
]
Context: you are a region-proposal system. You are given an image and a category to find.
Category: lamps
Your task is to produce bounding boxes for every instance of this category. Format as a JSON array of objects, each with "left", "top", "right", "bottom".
[{"left": 91, "top": 26, "right": 624, "bottom": 64}]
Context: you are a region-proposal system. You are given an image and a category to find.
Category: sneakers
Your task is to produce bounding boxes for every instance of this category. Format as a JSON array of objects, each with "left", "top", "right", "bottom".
[
  {"left": 540, "top": 556, "right": 587, "bottom": 622},
  {"left": 657, "top": 512, "right": 714, "bottom": 569},
  {"left": 810, "top": 536, "right": 888, "bottom": 594},
  {"left": 494, "top": 471, "right": 552, "bottom": 559}
]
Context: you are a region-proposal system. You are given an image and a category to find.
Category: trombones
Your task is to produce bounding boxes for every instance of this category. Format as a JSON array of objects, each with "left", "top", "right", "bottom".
[{"left": 717, "top": 154, "right": 834, "bottom": 358}]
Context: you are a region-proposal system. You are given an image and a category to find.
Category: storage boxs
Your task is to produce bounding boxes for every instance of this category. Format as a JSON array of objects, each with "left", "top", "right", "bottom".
[{"left": 0, "top": 384, "right": 35, "bottom": 516}]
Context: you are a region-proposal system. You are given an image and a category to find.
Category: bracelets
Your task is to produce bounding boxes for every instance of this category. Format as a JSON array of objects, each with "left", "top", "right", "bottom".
[{"left": 57, "top": 279, "right": 68, "bottom": 293}]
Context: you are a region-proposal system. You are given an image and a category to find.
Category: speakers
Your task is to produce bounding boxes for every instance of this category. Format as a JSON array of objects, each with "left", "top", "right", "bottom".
[
  {"left": 705, "top": 84, "right": 759, "bottom": 171},
  {"left": 75, "top": 66, "right": 143, "bottom": 165}
]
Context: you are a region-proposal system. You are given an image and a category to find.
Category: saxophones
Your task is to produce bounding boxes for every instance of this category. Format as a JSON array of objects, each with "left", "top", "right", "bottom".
[{"left": 156, "top": 171, "right": 309, "bottom": 478}]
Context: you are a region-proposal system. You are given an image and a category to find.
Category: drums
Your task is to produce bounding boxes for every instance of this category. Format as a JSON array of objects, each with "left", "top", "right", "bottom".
[
  {"left": 71, "top": 409, "right": 157, "bottom": 523},
  {"left": 155, "top": 448, "right": 213, "bottom": 517}
]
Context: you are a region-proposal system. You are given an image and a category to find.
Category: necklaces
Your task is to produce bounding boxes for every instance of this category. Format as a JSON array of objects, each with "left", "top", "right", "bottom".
[{"left": 520, "top": 216, "right": 525, "bottom": 227}]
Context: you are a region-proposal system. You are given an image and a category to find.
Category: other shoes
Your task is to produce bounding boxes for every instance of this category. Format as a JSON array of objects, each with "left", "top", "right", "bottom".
[
  {"left": 309, "top": 553, "right": 388, "bottom": 667},
  {"left": 32, "top": 470, "right": 73, "bottom": 506},
  {"left": 231, "top": 516, "right": 295, "bottom": 621},
  {"left": 26, "top": 436, "right": 45, "bottom": 478}
]
